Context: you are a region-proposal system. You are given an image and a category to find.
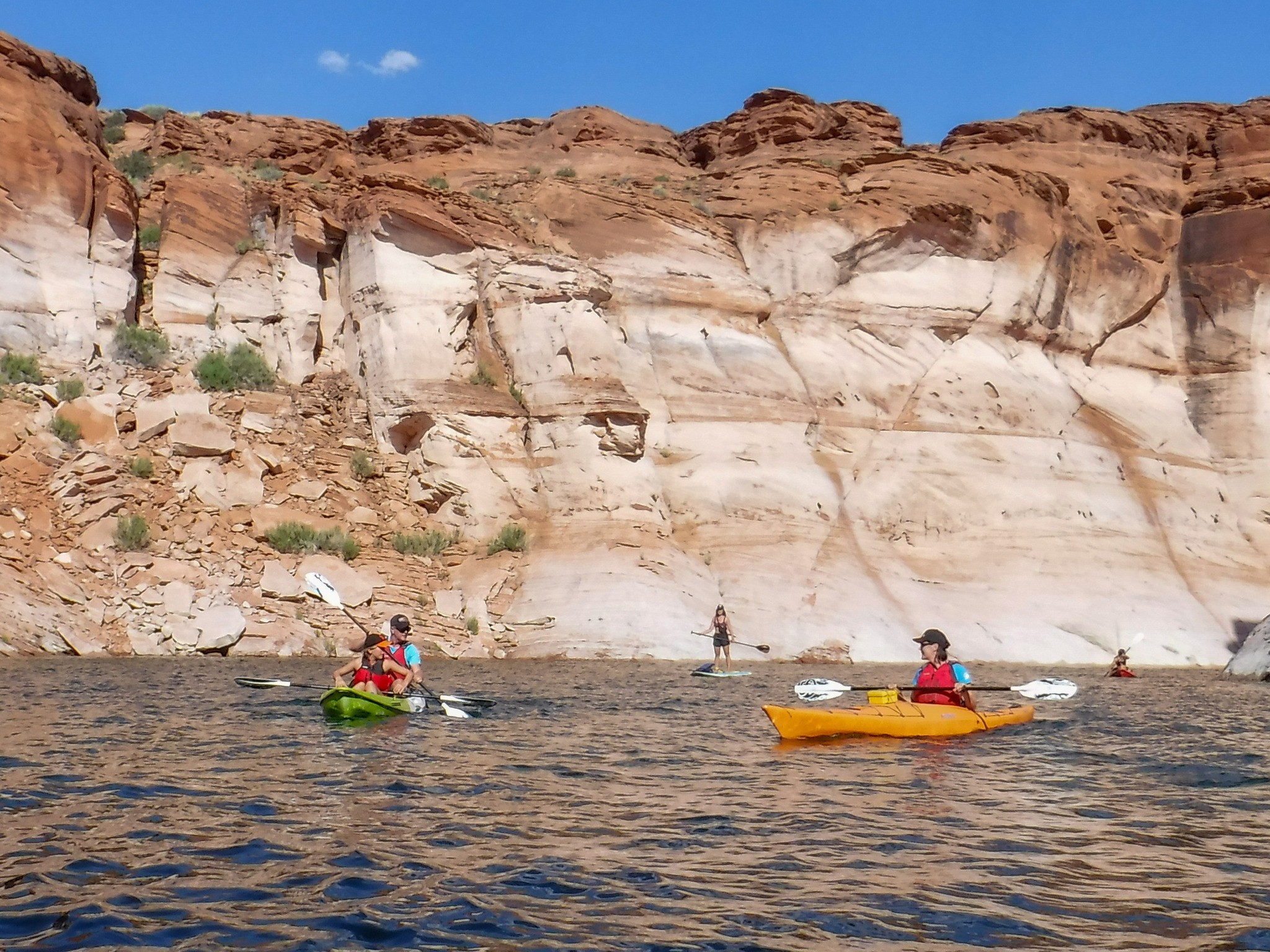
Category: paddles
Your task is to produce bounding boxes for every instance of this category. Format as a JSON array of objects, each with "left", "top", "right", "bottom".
[
  {"left": 690, "top": 632, "right": 772, "bottom": 653},
  {"left": 232, "top": 676, "right": 498, "bottom": 710},
  {"left": 1101, "top": 634, "right": 1143, "bottom": 678},
  {"left": 795, "top": 676, "right": 1078, "bottom": 702},
  {"left": 303, "top": 571, "right": 477, "bottom": 720}
]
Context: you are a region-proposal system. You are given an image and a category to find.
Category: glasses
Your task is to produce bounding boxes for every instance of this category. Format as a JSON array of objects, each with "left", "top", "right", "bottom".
[
  {"left": 396, "top": 627, "right": 411, "bottom": 633},
  {"left": 920, "top": 642, "right": 934, "bottom": 651}
]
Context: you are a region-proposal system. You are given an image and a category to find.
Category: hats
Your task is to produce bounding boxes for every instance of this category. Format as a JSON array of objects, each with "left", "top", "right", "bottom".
[
  {"left": 717, "top": 604, "right": 723, "bottom": 609},
  {"left": 364, "top": 634, "right": 393, "bottom": 649},
  {"left": 912, "top": 630, "right": 950, "bottom": 650},
  {"left": 391, "top": 614, "right": 410, "bottom": 630}
]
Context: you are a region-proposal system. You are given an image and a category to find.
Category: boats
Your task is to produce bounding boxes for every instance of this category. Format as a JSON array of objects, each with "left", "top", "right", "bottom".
[
  {"left": 761, "top": 689, "right": 1035, "bottom": 742},
  {"left": 319, "top": 685, "right": 426, "bottom": 725},
  {"left": 1108, "top": 666, "right": 1138, "bottom": 678}
]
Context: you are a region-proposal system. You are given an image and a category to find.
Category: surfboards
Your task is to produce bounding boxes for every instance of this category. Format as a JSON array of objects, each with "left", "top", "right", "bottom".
[{"left": 690, "top": 671, "right": 752, "bottom": 677}]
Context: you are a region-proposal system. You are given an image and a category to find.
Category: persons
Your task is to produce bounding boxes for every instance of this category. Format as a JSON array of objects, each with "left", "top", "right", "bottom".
[
  {"left": 333, "top": 614, "right": 424, "bottom": 697},
  {"left": 698, "top": 604, "right": 735, "bottom": 672},
  {"left": 888, "top": 629, "right": 977, "bottom": 712},
  {"left": 1109, "top": 649, "right": 1130, "bottom": 671}
]
